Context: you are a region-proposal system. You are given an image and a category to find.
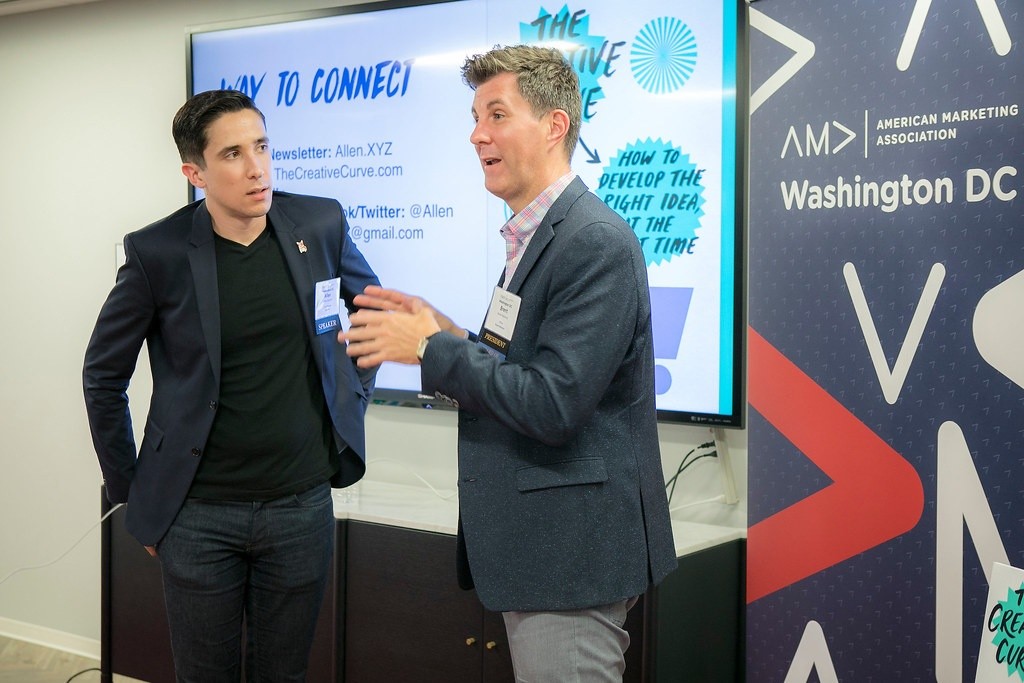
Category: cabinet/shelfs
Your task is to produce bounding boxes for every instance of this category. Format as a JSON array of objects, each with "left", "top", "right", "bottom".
[{"left": 101, "top": 479, "right": 747, "bottom": 683}]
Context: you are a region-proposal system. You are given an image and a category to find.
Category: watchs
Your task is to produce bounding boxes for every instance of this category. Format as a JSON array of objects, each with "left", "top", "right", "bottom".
[{"left": 416, "top": 332, "right": 439, "bottom": 364}]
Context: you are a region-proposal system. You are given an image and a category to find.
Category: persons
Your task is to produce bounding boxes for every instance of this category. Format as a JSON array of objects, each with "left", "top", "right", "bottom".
[
  {"left": 81, "top": 90, "right": 385, "bottom": 683},
  {"left": 339, "top": 42, "right": 679, "bottom": 683}
]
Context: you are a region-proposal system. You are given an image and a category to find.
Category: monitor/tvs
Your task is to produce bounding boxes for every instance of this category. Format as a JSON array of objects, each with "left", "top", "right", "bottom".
[{"left": 184, "top": 0, "right": 749, "bottom": 434}]
